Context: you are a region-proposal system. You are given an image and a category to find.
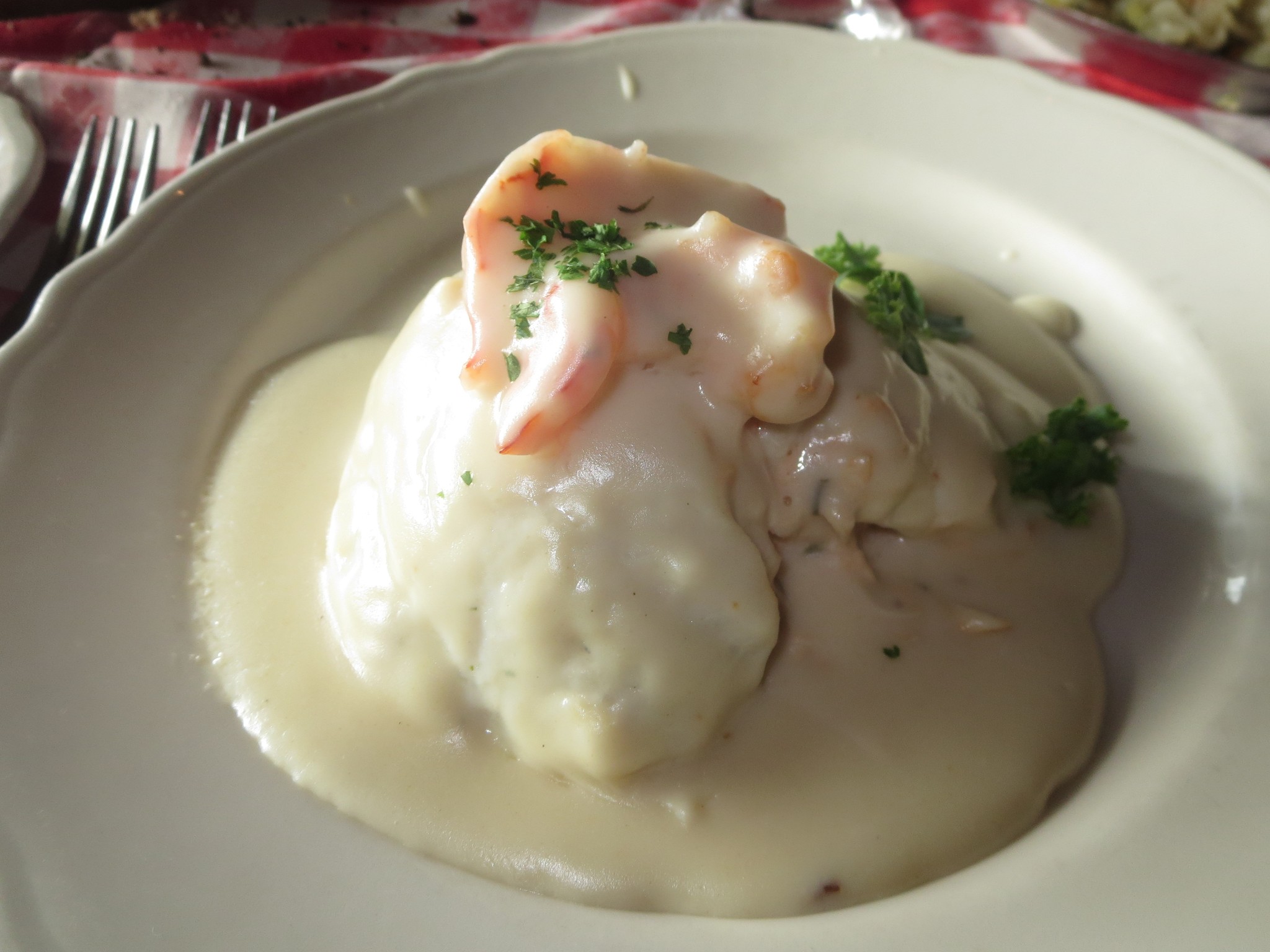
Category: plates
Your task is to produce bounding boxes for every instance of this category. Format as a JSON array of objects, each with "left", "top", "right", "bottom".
[
  {"left": 1, "top": 22, "right": 1266, "bottom": 950},
  {"left": 0, "top": 90, "right": 47, "bottom": 242}
]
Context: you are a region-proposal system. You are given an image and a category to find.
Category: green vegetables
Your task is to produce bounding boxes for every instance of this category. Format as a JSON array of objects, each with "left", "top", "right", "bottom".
[{"left": 496, "top": 162, "right": 1131, "bottom": 528}]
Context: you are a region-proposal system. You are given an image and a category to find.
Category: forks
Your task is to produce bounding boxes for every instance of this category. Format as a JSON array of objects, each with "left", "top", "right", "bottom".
[
  {"left": 25, "top": 112, "right": 162, "bottom": 322},
  {"left": 184, "top": 98, "right": 277, "bottom": 174}
]
